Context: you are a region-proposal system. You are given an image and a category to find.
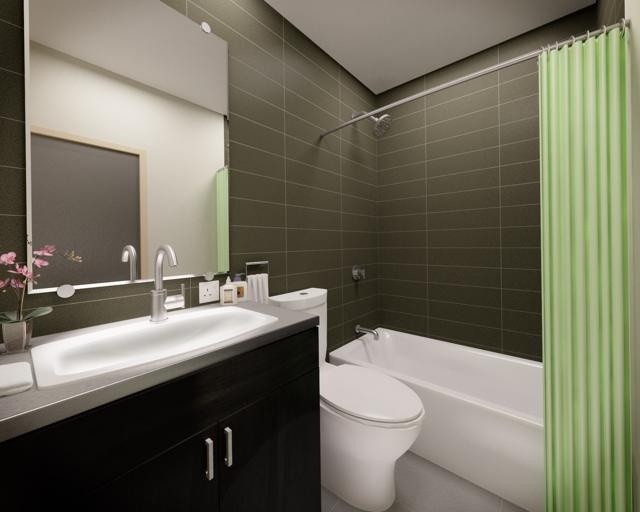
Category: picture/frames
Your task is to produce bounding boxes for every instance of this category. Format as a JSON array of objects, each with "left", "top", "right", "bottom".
[{"left": 22, "top": 0, "right": 234, "bottom": 298}]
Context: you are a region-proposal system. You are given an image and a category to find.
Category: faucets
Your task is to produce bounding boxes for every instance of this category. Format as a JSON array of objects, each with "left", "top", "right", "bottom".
[
  {"left": 149, "top": 244, "right": 185, "bottom": 322},
  {"left": 120, "top": 244, "right": 138, "bottom": 280},
  {"left": 355, "top": 323, "right": 379, "bottom": 341}
]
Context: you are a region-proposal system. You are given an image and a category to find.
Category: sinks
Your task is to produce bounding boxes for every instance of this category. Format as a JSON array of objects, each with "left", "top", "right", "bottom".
[{"left": 30, "top": 306, "right": 279, "bottom": 391}]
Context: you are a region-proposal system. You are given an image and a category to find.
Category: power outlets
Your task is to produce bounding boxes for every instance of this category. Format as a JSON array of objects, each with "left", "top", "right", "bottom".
[{"left": 197, "top": 279, "right": 222, "bottom": 303}]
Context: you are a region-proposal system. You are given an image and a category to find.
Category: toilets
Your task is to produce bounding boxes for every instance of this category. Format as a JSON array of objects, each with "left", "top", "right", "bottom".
[{"left": 267, "top": 287, "right": 425, "bottom": 512}]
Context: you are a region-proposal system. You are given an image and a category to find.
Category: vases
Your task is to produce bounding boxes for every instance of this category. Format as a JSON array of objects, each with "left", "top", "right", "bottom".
[{"left": 1, "top": 320, "right": 36, "bottom": 353}]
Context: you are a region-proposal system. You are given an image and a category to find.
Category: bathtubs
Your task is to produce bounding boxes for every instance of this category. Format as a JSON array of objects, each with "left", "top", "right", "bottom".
[{"left": 328, "top": 327, "right": 545, "bottom": 512}]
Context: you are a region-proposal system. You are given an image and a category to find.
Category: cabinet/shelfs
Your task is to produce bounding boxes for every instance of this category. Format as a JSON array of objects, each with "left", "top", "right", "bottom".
[{"left": 1, "top": 325, "right": 329, "bottom": 512}]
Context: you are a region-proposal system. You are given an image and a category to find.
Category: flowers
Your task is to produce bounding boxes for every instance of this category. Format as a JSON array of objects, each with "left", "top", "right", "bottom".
[{"left": 0, "top": 245, "right": 63, "bottom": 320}]
